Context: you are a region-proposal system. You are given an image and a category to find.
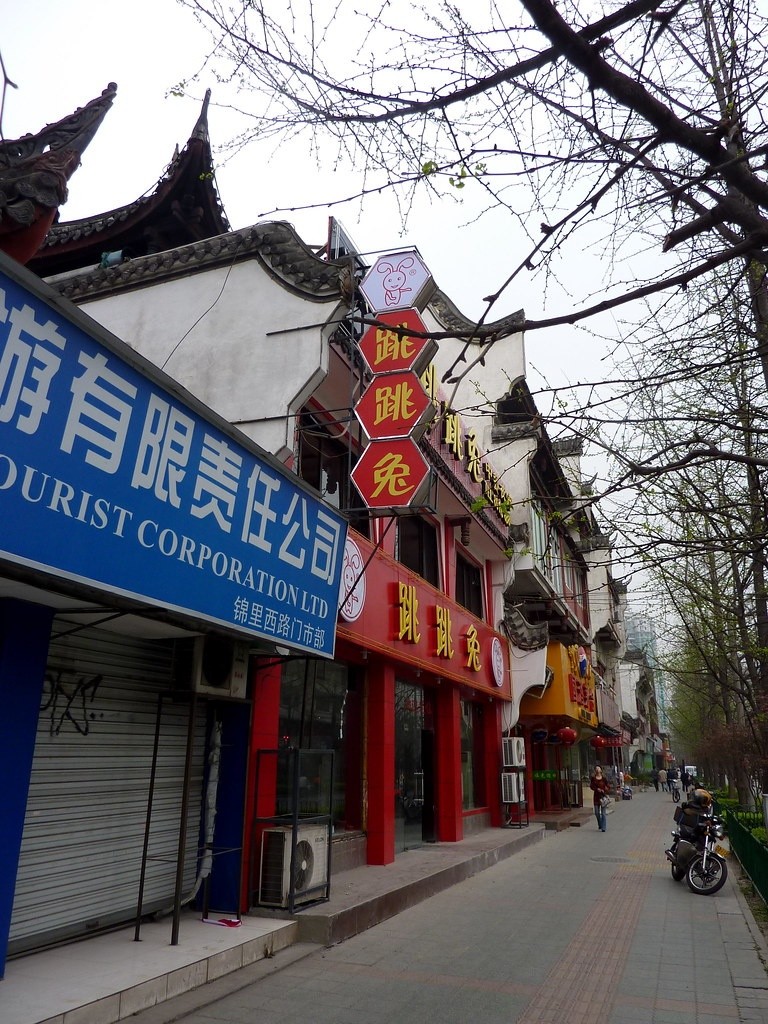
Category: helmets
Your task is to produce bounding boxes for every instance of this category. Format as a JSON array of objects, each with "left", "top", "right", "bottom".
[{"left": 692, "top": 789, "right": 712, "bottom": 807}]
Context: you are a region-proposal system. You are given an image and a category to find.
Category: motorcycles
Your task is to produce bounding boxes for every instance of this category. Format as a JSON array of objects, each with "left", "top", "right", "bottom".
[
  {"left": 665, "top": 797, "right": 733, "bottom": 897},
  {"left": 669, "top": 778, "right": 681, "bottom": 803}
]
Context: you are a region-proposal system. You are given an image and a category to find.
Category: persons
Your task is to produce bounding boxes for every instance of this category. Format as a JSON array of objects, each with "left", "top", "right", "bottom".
[
  {"left": 651, "top": 766, "right": 659, "bottom": 792},
  {"left": 658, "top": 769, "right": 667, "bottom": 791},
  {"left": 680, "top": 769, "right": 688, "bottom": 792},
  {"left": 666, "top": 767, "right": 682, "bottom": 792},
  {"left": 624, "top": 771, "right": 633, "bottom": 787},
  {"left": 590, "top": 765, "right": 611, "bottom": 832},
  {"left": 686, "top": 769, "right": 692, "bottom": 786}
]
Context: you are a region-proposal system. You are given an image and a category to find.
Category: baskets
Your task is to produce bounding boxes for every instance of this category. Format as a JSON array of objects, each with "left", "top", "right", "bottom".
[{"left": 673, "top": 781, "right": 682, "bottom": 789}]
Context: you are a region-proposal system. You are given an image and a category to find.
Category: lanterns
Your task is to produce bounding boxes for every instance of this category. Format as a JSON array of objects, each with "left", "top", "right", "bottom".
[
  {"left": 590, "top": 735, "right": 605, "bottom": 747},
  {"left": 557, "top": 727, "right": 577, "bottom": 745}
]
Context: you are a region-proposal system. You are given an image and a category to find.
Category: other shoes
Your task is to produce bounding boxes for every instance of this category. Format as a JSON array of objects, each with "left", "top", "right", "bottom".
[
  {"left": 602, "top": 829, "right": 605, "bottom": 832},
  {"left": 599, "top": 826, "right": 601, "bottom": 829}
]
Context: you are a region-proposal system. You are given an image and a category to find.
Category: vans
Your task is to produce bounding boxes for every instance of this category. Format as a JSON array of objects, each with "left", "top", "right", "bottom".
[{"left": 685, "top": 766, "right": 697, "bottom": 777}]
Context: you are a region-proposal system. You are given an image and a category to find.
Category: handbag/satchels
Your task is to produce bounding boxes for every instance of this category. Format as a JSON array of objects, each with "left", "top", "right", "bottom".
[
  {"left": 664, "top": 783, "right": 668, "bottom": 791},
  {"left": 600, "top": 791, "right": 612, "bottom": 808}
]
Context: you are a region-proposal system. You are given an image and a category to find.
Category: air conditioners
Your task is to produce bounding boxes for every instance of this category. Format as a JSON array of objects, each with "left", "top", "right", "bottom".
[
  {"left": 502, "top": 773, "right": 525, "bottom": 803},
  {"left": 182, "top": 634, "right": 249, "bottom": 698},
  {"left": 257, "top": 823, "right": 328, "bottom": 908},
  {"left": 502, "top": 737, "right": 526, "bottom": 767}
]
